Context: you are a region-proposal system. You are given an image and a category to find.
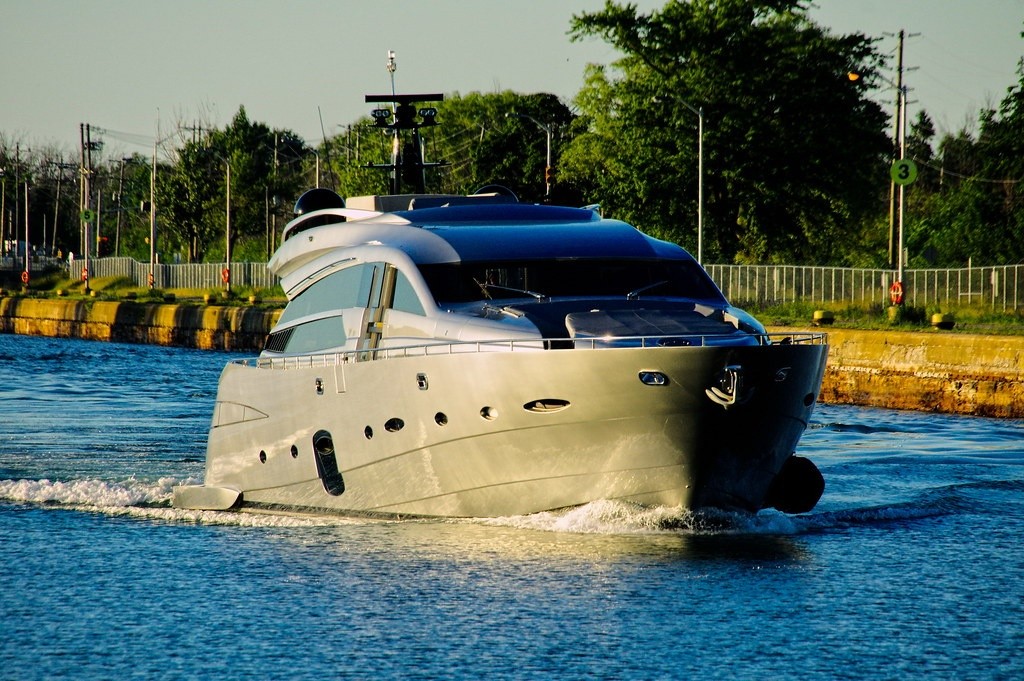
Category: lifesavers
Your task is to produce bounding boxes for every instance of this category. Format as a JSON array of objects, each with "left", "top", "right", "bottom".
[
  {"left": 21, "top": 271, "right": 31, "bottom": 284},
  {"left": 82, "top": 268, "right": 89, "bottom": 282},
  {"left": 890, "top": 282, "right": 905, "bottom": 304},
  {"left": 149, "top": 275, "right": 153, "bottom": 285},
  {"left": 222, "top": 269, "right": 231, "bottom": 282}
]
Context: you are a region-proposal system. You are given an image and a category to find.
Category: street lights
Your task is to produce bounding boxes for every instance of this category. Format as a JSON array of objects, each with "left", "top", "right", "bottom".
[
  {"left": 848, "top": 65, "right": 905, "bottom": 297},
  {"left": 650, "top": 91, "right": 705, "bottom": 268},
  {"left": 504, "top": 109, "right": 556, "bottom": 203},
  {"left": 193, "top": 146, "right": 234, "bottom": 299},
  {"left": 279, "top": 132, "right": 321, "bottom": 190}
]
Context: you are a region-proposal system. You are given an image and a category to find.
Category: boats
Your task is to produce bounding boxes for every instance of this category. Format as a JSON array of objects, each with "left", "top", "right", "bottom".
[{"left": 175, "top": 49, "right": 830, "bottom": 539}]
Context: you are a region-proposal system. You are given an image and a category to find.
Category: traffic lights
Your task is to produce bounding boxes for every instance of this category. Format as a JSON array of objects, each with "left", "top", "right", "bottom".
[{"left": 546, "top": 168, "right": 554, "bottom": 187}]
